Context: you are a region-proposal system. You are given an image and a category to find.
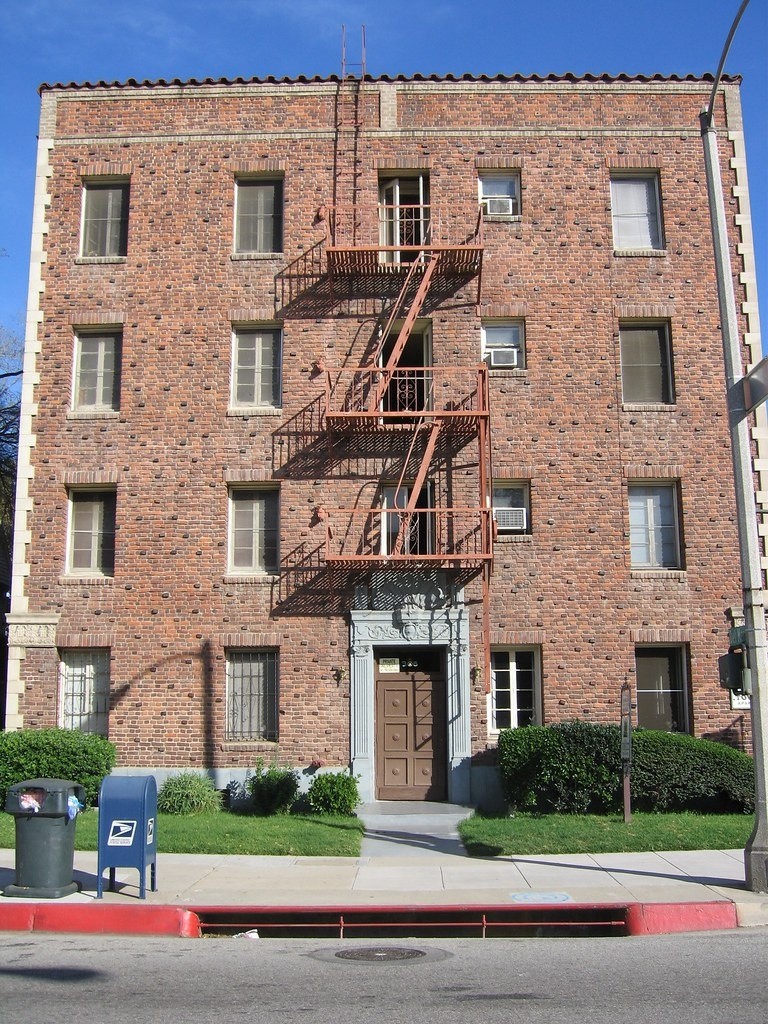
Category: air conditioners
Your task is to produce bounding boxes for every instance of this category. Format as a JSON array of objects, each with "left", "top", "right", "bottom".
[
  {"left": 491, "top": 348, "right": 518, "bottom": 368},
  {"left": 492, "top": 507, "right": 528, "bottom": 531},
  {"left": 487, "top": 198, "right": 513, "bottom": 215}
]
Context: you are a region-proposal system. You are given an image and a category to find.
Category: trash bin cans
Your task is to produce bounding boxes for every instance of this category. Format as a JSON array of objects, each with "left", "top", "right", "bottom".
[{"left": 2, "top": 778, "right": 87, "bottom": 899}]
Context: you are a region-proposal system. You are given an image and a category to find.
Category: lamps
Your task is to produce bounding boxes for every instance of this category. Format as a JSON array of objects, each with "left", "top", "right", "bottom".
[
  {"left": 335, "top": 666, "right": 346, "bottom": 686},
  {"left": 474, "top": 660, "right": 482, "bottom": 681}
]
